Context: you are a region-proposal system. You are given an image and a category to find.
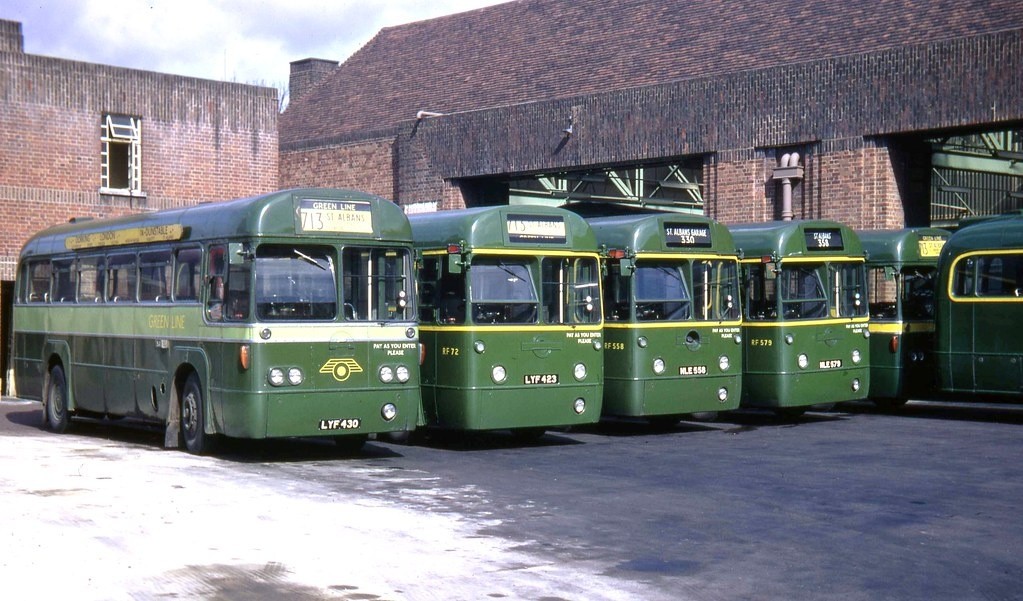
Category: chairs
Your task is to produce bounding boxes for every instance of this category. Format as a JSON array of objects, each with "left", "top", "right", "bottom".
[{"left": 28, "top": 264, "right": 360, "bottom": 319}]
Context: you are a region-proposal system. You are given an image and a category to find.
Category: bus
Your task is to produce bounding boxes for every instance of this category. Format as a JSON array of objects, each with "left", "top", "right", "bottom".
[
  {"left": 13, "top": 188, "right": 422, "bottom": 455},
  {"left": 855, "top": 228, "right": 956, "bottom": 409},
  {"left": 933, "top": 209, "right": 1023, "bottom": 405},
  {"left": 580, "top": 212, "right": 747, "bottom": 431},
  {"left": 726, "top": 220, "right": 871, "bottom": 417},
  {"left": 402, "top": 205, "right": 609, "bottom": 443}
]
